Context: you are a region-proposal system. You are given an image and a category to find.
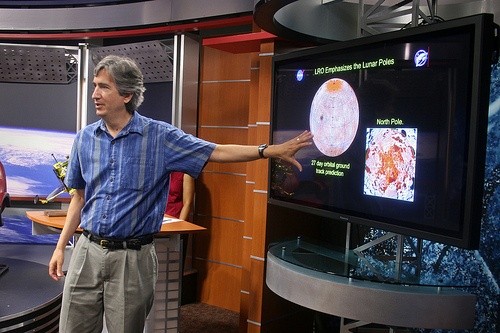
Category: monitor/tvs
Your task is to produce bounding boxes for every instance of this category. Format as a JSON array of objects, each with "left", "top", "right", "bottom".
[{"left": 268, "top": 13, "right": 495, "bottom": 251}]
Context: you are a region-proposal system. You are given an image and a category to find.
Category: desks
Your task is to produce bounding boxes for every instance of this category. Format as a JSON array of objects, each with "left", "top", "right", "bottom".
[
  {"left": 265, "top": 232, "right": 477, "bottom": 333},
  {"left": 25, "top": 209, "right": 207, "bottom": 333}
]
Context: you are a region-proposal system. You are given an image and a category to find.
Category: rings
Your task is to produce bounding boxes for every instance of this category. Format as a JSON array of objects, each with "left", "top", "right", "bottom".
[{"left": 297, "top": 138, "right": 299, "bottom": 141}]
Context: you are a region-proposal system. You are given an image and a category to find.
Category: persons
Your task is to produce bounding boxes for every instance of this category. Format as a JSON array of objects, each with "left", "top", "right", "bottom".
[
  {"left": 165, "top": 171, "right": 195, "bottom": 221},
  {"left": 48, "top": 55, "right": 315, "bottom": 333}
]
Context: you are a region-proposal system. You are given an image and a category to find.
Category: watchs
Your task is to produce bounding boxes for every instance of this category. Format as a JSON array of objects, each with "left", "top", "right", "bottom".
[{"left": 258, "top": 144, "right": 268, "bottom": 159}]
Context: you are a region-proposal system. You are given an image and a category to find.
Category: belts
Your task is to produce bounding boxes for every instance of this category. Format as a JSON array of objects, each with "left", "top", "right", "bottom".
[{"left": 81, "top": 229, "right": 155, "bottom": 250}]
